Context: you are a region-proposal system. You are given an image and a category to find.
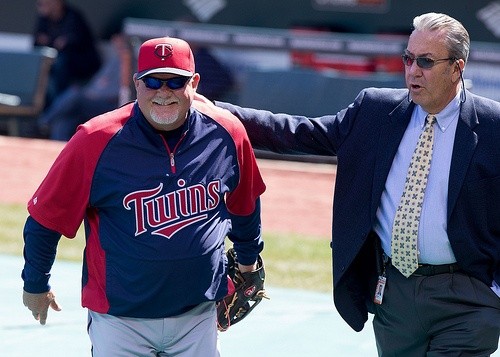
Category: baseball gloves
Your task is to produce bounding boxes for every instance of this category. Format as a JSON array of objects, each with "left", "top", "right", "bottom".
[{"left": 216, "top": 248, "right": 269, "bottom": 334}]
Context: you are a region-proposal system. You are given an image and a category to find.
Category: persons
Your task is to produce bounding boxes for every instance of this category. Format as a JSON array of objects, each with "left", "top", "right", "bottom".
[
  {"left": 29, "top": 0, "right": 235, "bottom": 142},
  {"left": 20, "top": 37, "right": 268, "bottom": 357},
  {"left": 194, "top": 10, "right": 500, "bottom": 357}
]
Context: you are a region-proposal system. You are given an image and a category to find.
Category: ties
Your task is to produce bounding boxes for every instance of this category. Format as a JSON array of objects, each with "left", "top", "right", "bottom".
[{"left": 389, "top": 113, "right": 434, "bottom": 279}]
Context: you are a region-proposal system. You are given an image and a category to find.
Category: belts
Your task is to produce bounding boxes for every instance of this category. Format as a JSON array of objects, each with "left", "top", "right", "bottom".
[{"left": 387, "top": 261, "right": 458, "bottom": 276}]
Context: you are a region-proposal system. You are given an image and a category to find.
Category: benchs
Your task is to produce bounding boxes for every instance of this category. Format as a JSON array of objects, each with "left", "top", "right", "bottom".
[{"left": 0, "top": 43, "right": 58, "bottom": 136}]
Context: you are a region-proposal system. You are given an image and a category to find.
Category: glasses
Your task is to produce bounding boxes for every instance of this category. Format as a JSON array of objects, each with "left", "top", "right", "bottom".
[
  {"left": 139, "top": 75, "right": 191, "bottom": 89},
  {"left": 401, "top": 48, "right": 455, "bottom": 69}
]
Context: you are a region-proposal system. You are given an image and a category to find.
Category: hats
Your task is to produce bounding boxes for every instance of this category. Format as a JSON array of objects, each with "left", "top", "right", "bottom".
[{"left": 136, "top": 36, "right": 196, "bottom": 80}]
